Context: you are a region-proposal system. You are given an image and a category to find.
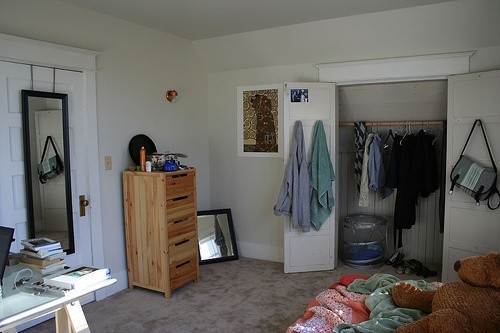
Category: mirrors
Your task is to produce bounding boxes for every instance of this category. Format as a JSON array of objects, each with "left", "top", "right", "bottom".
[
  {"left": 197, "top": 208, "right": 239, "bottom": 264},
  {"left": 22, "top": 89, "right": 75, "bottom": 256}
]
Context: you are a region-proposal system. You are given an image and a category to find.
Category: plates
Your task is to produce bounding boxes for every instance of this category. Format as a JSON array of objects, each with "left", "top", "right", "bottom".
[{"left": 127, "top": 133, "right": 157, "bottom": 164}]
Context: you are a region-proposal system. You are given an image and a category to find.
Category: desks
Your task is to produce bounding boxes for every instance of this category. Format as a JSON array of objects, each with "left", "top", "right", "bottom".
[{"left": 0, "top": 268, "right": 118, "bottom": 333}]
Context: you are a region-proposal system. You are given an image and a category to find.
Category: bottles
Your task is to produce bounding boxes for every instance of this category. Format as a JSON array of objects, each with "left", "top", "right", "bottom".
[{"left": 140, "top": 146, "right": 146, "bottom": 173}]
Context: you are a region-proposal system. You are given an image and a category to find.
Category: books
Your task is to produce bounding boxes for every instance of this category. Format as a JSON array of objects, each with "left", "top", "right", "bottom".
[{"left": 19, "top": 237, "right": 113, "bottom": 297}]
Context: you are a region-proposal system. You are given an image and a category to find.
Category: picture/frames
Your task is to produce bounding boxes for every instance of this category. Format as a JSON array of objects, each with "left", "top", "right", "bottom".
[{"left": 236, "top": 84, "right": 283, "bottom": 159}]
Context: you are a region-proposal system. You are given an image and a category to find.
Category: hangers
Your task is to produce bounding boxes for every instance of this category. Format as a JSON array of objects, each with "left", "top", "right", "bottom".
[{"left": 370, "top": 121, "right": 445, "bottom": 147}]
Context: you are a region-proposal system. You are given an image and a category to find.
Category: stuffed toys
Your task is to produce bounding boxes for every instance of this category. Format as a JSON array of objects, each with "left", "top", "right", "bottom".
[{"left": 391, "top": 251, "right": 500, "bottom": 333}]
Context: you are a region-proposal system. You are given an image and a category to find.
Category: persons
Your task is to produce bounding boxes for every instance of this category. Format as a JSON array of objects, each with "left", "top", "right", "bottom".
[{"left": 292, "top": 91, "right": 306, "bottom": 102}]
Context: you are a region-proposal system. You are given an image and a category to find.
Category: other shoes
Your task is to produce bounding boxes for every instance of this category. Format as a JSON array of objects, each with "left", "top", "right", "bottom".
[
  {"left": 396, "top": 261, "right": 416, "bottom": 275},
  {"left": 386, "top": 249, "right": 404, "bottom": 268}
]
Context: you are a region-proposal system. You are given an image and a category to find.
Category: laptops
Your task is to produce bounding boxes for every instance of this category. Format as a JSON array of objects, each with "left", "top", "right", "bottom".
[{"left": 0, "top": 226, "right": 15, "bottom": 286}]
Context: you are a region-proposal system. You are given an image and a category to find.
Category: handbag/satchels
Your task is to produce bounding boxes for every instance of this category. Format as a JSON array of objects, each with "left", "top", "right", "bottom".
[
  {"left": 36, "top": 137, "right": 64, "bottom": 185},
  {"left": 449, "top": 120, "right": 497, "bottom": 207}
]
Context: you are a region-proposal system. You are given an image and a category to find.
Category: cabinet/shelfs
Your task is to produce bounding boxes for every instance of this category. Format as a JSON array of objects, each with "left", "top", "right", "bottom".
[{"left": 121, "top": 169, "right": 200, "bottom": 298}]
